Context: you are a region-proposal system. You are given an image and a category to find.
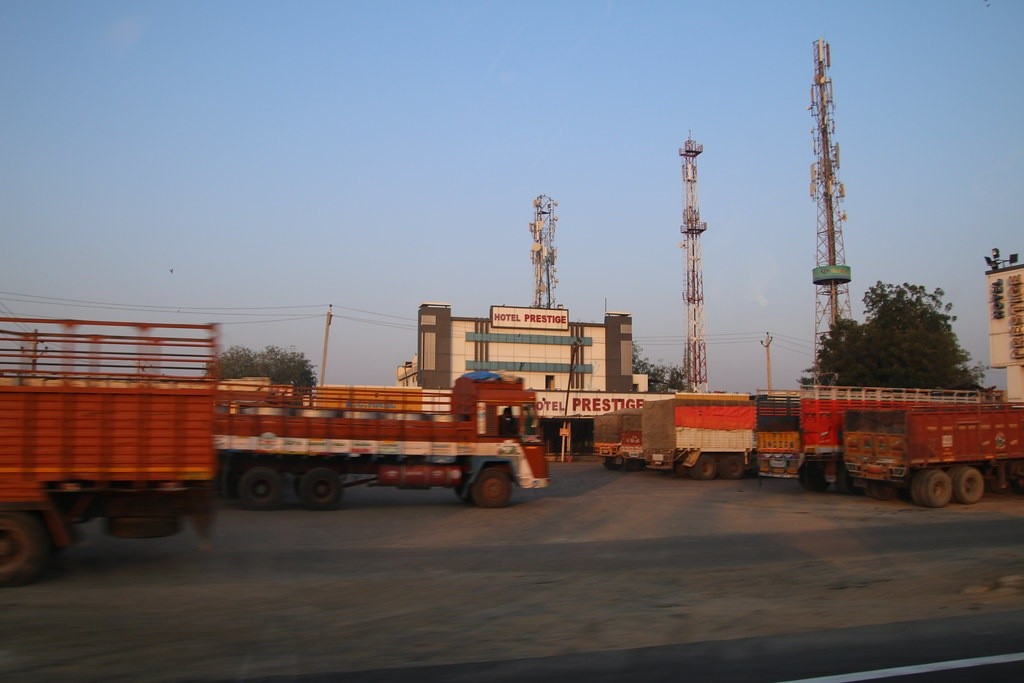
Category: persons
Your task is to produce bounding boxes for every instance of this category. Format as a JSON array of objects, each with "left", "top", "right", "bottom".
[{"left": 498, "top": 408, "right": 515, "bottom": 436}]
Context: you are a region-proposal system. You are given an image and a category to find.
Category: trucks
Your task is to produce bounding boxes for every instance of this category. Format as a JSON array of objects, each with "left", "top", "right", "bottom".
[
  {"left": 0, "top": 317, "right": 221, "bottom": 589},
  {"left": 755, "top": 396, "right": 945, "bottom": 495},
  {"left": 643, "top": 398, "right": 800, "bottom": 480},
  {"left": 217, "top": 368, "right": 550, "bottom": 511},
  {"left": 592, "top": 410, "right": 645, "bottom": 472}
]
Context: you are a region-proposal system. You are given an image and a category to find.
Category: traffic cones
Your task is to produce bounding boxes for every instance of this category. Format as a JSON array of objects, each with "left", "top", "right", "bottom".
[{"left": 567, "top": 454, "right": 572, "bottom": 462}]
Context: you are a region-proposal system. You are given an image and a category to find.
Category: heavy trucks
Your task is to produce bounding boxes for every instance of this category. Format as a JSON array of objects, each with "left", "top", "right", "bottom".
[{"left": 842, "top": 406, "right": 1024, "bottom": 507}]
